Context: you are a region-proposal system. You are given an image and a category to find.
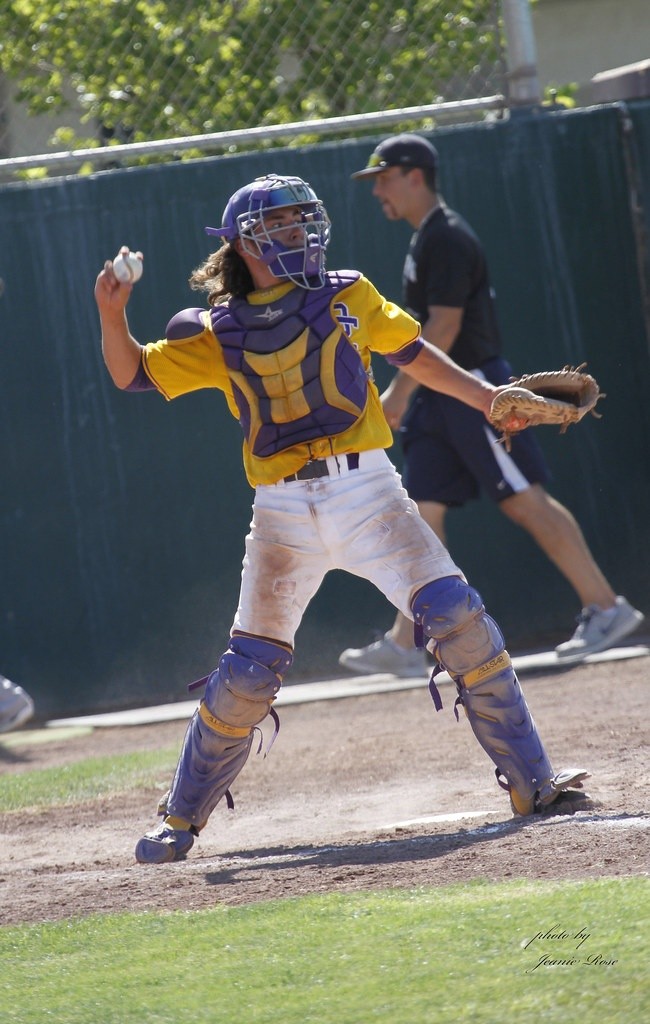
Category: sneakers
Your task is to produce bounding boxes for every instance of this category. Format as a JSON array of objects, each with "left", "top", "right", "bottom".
[
  {"left": 554, "top": 595, "right": 644, "bottom": 658},
  {"left": 338, "top": 631, "right": 430, "bottom": 677}
]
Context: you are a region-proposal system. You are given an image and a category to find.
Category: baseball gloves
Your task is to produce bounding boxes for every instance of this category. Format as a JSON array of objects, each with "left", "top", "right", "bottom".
[{"left": 489, "top": 362, "right": 606, "bottom": 454}]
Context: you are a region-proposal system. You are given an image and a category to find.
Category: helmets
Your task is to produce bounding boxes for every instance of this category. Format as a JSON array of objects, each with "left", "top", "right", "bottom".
[{"left": 204, "top": 173, "right": 332, "bottom": 291}]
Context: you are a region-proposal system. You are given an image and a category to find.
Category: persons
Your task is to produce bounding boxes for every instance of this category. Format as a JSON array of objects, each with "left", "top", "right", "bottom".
[
  {"left": 93, "top": 172, "right": 594, "bottom": 864},
  {"left": 337, "top": 134, "right": 646, "bottom": 680}
]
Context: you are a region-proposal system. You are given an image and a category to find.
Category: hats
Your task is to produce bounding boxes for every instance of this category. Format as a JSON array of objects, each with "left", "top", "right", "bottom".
[{"left": 350, "top": 134, "right": 438, "bottom": 181}]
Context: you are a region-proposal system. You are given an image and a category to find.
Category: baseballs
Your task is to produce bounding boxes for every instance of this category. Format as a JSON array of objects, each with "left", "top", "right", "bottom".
[{"left": 112, "top": 252, "right": 143, "bottom": 283}]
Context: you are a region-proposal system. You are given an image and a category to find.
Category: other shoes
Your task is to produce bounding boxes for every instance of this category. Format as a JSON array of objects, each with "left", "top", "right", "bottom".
[
  {"left": 0, "top": 674, "right": 34, "bottom": 733},
  {"left": 135, "top": 826, "right": 194, "bottom": 861},
  {"left": 510, "top": 788, "right": 588, "bottom": 817}
]
